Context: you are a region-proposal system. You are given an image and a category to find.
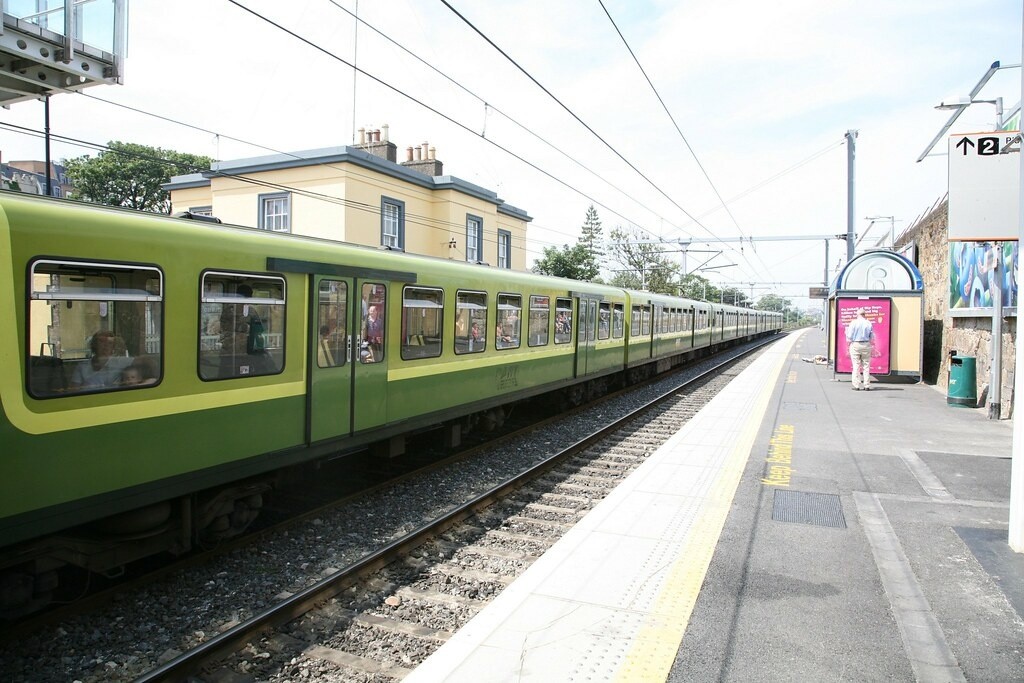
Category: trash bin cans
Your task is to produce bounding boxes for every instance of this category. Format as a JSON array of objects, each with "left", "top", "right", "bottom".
[{"left": 947, "top": 355, "right": 978, "bottom": 408}]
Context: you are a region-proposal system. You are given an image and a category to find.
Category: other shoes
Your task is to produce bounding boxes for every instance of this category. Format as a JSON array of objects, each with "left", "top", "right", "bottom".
[
  {"left": 863, "top": 387, "right": 869, "bottom": 390},
  {"left": 852, "top": 387, "right": 859, "bottom": 390}
]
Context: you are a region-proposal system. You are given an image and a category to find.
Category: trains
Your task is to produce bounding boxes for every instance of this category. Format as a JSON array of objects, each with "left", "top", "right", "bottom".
[{"left": 1, "top": 185, "right": 782, "bottom": 628}]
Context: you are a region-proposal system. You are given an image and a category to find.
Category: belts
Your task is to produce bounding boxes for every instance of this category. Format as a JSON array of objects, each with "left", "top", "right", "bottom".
[{"left": 852, "top": 340, "right": 868, "bottom": 342}]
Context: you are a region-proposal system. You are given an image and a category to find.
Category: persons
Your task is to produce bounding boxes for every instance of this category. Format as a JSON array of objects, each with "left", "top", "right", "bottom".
[
  {"left": 529, "top": 294, "right": 715, "bottom": 346},
  {"left": 326, "top": 317, "right": 346, "bottom": 350},
  {"left": 496, "top": 320, "right": 504, "bottom": 341},
  {"left": 66, "top": 330, "right": 153, "bottom": 395},
  {"left": 216, "top": 284, "right": 263, "bottom": 376},
  {"left": 366, "top": 306, "right": 383, "bottom": 354},
  {"left": 455, "top": 313, "right": 465, "bottom": 336},
  {"left": 115, "top": 361, "right": 156, "bottom": 387},
  {"left": 319, "top": 326, "right": 333, "bottom": 343},
  {"left": 360, "top": 294, "right": 368, "bottom": 336},
  {"left": 473, "top": 326, "right": 480, "bottom": 340},
  {"left": 843, "top": 307, "right": 875, "bottom": 390},
  {"left": 472, "top": 321, "right": 482, "bottom": 342}
]
